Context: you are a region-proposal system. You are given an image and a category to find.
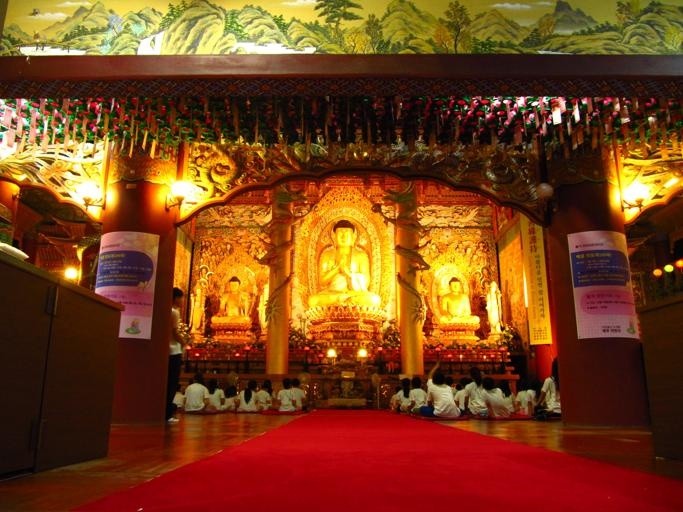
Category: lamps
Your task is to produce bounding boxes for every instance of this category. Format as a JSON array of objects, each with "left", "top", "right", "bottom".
[
  {"left": 166, "top": 179, "right": 198, "bottom": 212},
  {"left": 74, "top": 183, "right": 106, "bottom": 210},
  {"left": 619, "top": 184, "right": 647, "bottom": 211}
]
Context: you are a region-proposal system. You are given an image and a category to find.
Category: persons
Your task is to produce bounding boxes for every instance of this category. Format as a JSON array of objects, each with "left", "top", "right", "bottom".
[
  {"left": 166, "top": 287, "right": 185, "bottom": 422},
  {"left": 211, "top": 276, "right": 252, "bottom": 324},
  {"left": 440, "top": 276, "right": 480, "bottom": 324},
  {"left": 389, "top": 355, "right": 562, "bottom": 421},
  {"left": 308, "top": 220, "right": 381, "bottom": 308},
  {"left": 172, "top": 373, "right": 307, "bottom": 412}
]
